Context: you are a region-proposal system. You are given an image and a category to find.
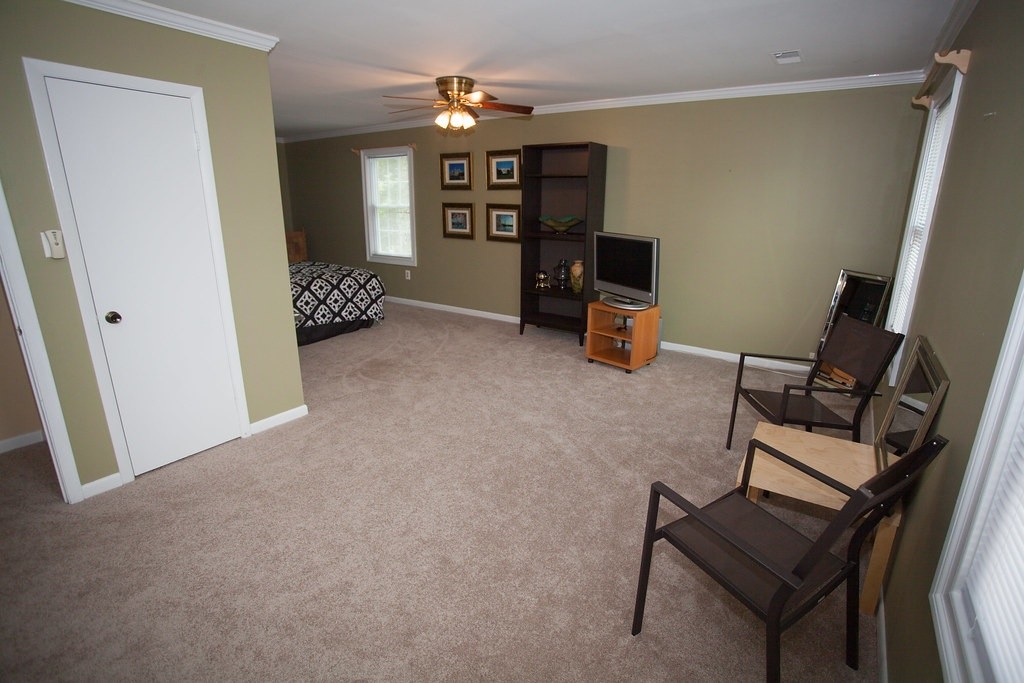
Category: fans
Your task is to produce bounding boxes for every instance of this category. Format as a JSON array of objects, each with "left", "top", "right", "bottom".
[{"left": 381, "top": 77, "right": 535, "bottom": 122}]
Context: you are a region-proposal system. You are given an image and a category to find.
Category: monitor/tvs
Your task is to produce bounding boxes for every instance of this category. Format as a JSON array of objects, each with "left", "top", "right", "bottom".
[{"left": 594, "top": 231, "right": 660, "bottom": 310}]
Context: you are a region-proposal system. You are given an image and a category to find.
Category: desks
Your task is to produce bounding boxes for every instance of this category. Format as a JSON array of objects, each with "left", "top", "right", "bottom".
[{"left": 736, "top": 421, "right": 902, "bottom": 616}]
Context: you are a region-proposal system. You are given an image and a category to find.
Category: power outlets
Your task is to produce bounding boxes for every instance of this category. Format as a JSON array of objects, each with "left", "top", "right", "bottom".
[{"left": 404, "top": 270, "right": 411, "bottom": 280}]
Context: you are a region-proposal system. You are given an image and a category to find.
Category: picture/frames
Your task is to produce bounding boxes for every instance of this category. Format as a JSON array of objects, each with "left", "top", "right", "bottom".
[
  {"left": 440, "top": 152, "right": 473, "bottom": 191},
  {"left": 486, "top": 203, "right": 521, "bottom": 242},
  {"left": 486, "top": 149, "right": 522, "bottom": 190},
  {"left": 442, "top": 203, "right": 476, "bottom": 240}
]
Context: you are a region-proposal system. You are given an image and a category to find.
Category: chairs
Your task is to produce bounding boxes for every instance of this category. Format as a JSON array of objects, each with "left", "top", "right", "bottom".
[
  {"left": 726, "top": 313, "right": 905, "bottom": 451},
  {"left": 632, "top": 433, "right": 949, "bottom": 683}
]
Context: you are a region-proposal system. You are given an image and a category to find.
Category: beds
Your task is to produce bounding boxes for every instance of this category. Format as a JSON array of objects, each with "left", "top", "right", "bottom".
[{"left": 285, "top": 231, "right": 385, "bottom": 348}]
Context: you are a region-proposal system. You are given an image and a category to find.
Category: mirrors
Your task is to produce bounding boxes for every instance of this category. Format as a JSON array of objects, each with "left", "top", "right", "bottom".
[{"left": 873, "top": 336, "right": 950, "bottom": 473}]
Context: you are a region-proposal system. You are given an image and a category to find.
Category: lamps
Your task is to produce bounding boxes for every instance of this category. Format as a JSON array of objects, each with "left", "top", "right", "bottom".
[{"left": 434, "top": 105, "right": 477, "bottom": 131}]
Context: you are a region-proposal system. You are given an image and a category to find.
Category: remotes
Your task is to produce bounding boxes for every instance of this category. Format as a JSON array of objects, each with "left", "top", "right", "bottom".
[{"left": 613, "top": 296, "right": 633, "bottom": 304}]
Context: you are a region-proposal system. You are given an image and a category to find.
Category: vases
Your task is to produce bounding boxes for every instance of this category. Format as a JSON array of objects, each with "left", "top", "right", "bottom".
[
  {"left": 569, "top": 260, "right": 584, "bottom": 294},
  {"left": 553, "top": 258, "right": 569, "bottom": 290}
]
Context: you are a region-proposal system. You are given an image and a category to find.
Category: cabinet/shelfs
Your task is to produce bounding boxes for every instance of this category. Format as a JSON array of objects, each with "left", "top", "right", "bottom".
[
  {"left": 586, "top": 301, "right": 661, "bottom": 373},
  {"left": 520, "top": 141, "right": 607, "bottom": 345}
]
